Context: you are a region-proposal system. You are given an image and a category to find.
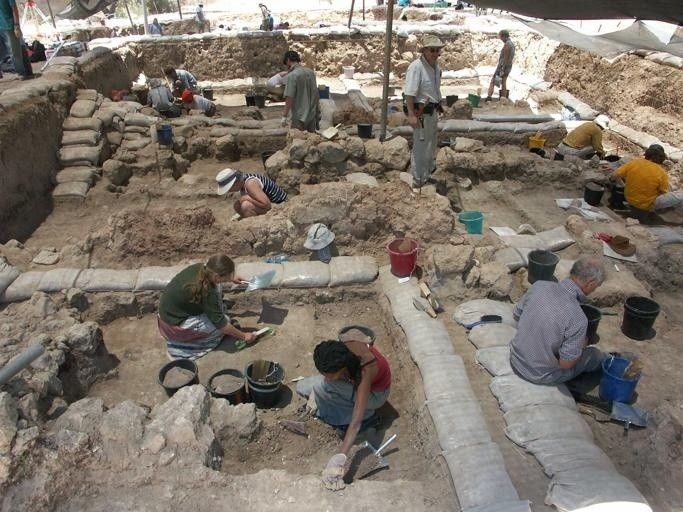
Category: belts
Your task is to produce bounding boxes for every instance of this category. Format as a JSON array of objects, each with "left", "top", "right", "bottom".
[{"left": 562, "top": 140, "right": 569, "bottom": 146}]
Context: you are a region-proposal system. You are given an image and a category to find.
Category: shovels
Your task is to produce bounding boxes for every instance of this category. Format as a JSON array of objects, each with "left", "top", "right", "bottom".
[
  {"left": 579, "top": 407, "right": 611, "bottom": 421},
  {"left": 610, "top": 400, "right": 648, "bottom": 431},
  {"left": 346, "top": 433, "right": 397, "bottom": 480}
]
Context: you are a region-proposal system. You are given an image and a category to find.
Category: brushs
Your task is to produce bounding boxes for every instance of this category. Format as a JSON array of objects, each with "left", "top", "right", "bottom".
[
  {"left": 420, "top": 282, "right": 445, "bottom": 313},
  {"left": 465, "top": 315, "right": 502, "bottom": 328}
]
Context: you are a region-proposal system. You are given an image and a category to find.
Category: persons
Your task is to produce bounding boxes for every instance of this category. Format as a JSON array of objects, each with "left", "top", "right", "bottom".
[
  {"left": 157, "top": 254, "right": 257, "bottom": 362},
  {"left": 258, "top": 2, "right": 289, "bottom": 31},
  {"left": 195, "top": 2, "right": 206, "bottom": 33},
  {"left": 150, "top": 17, "right": 163, "bottom": 37},
  {"left": 557, "top": 114, "right": 609, "bottom": 160},
  {"left": 608, "top": 143, "right": 683, "bottom": 213},
  {"left": 215, "top": 169, "right": 288, "bottom": 219},
  {"left": 280, "top": 50, "right": 322, "bottom": 133},
  {"left": 145, "top": 65, "right": 216, "bottom": 118},
  {"left": 0, "top": 0, "right": 41, "bottom": 80},
  {"left": 399, "top": 0, "right": 413, "bottom": 7},
  {"left": 295, "top": 339, "right": 392, "bottom": 492},
  {"left": 485, "top": 30, "right": 515, "bottom": 102},
  {"left": 403, "top": 34, "right": 446, "bottom": 189},
  {"left": 508, "top": 256, "right": 611, "bottom": 401}
]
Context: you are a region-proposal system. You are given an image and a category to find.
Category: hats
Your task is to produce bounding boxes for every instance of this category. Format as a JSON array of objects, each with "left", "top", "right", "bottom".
[
  {"left": 303, "top": 223, "right": 335, "bottom": 251},
  {"left": 416, "top": 34, "right": 445, "bottom": 52},
  {"left": 150, "top": 78, "right": 162, "bottom": 89},
  {"left": 650, "top": 144, "right": 664, "bottom": 153},
  {"left": 216, "top": 168, "right": 243, "bottom": 196},
  {"left": 610, "top": 235, "right": 636, "bottom": 257},
  {"left": 593, "top": 113, "right": 609, "bottom": 130}
]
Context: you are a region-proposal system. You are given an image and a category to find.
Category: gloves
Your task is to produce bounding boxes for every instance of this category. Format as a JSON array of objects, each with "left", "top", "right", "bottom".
[{"left": 281, "top": 117, "right": 288, "bottom": 127}]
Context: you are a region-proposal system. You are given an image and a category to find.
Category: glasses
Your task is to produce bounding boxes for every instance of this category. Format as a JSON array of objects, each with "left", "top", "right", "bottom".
[{"left": 430, "top": 48, "right": 439, "bottom": 53}]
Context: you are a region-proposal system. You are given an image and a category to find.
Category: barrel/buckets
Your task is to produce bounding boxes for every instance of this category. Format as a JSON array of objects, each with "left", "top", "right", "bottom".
[
  {"left": 203, "top": 88, "right": 213, "bottom": 101},
  {"left": 459, "top": 211, "right": 484, "bottom": 233},
  {"left": 599, "top": 356, "right": 640, "bottom": 403},
  {"left": 499, "top": 90, "right": 509, "bottom": 98},
  {"left": 446, "top": 95, "right": 458, "bottom": 107},
  {"left": 621, "top": 296, "right": 661, "bottom": 340},
  {"left": 157, "top": 358, "right": 198, "bottom": 398},
  {"left": 468, "top": 93, "right": 480, "bottom": 108},
  {"left": 245, "top": 357, "right": 286, "bottom": 405},
  {"left": 529, "top": 136, "right": 546, "bottom": 151},
  {"left": 156, "top": 125, "right": 173, "bottom": 145},
  {"left": 386, "top": 237, "right": 417, "bottom": 277},
  {"left": 339, "top": 325, "right": 377, "bottom": 346},
  {"left": 318, "top": 87, "right": 329, "bottom": 99},
  {"left": 585, "top": 180, "right": 605, "bottom": 207},
  {"left": 207, "top": 368, "right": 247, "bottom": 404},
  {"left": 580, "top": 304, "right": 602, "bottom": 335},
  {"left": 246, "top": 95, "right": 265, "bottom": 108},
  {"left": 344, "top": 66, "right": 355, "bottom": 79},
  {"left": 357, "top": 123, "right": 372, "bottom": 139},
  {"left": 528, "top": 250, "right": 560, "bottom": 283}
]
